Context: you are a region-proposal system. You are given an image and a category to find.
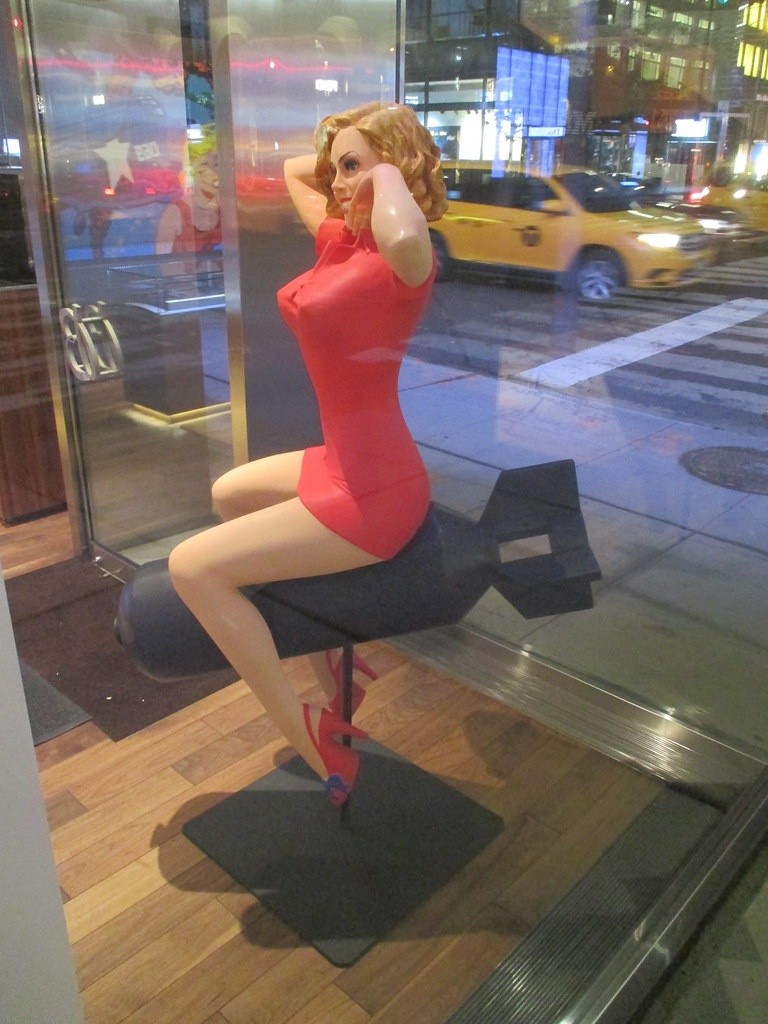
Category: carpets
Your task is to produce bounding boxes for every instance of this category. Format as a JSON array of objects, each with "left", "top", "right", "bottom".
[
  {"left": 5, "top": 553, "right": 243, "bottom": 741},
  {"left": 15, "top": 655, "right": 93, "bottom": 748}
]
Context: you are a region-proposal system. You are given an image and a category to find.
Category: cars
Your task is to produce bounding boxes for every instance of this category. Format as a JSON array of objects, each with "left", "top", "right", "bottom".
[
  {"left": 685, "top": 160, "right": 768, "bottom": 234},
  {"left": 423, "top": 159, "right": 746, "bottom": 307}
]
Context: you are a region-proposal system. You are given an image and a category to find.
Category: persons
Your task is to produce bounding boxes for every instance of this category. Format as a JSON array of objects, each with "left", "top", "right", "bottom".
[{"left": 168, "top": 103, "right": 450, "bottom": 803}]
